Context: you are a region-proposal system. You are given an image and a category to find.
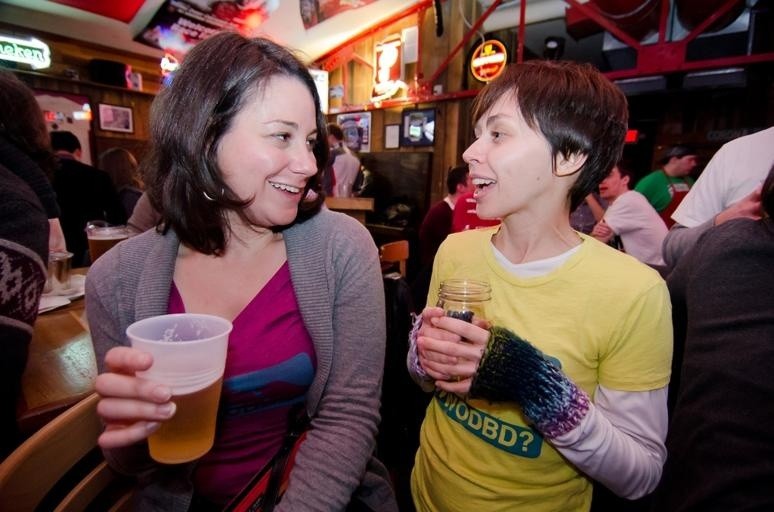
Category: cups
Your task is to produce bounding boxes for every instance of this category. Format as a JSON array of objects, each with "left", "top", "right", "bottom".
[
  {"left": 85, "top": 222, "right": 133, "bottom": 267},
  {"left": 125, "top": 314, "right": 233, "bottom": 466},
  {"left": 51, "top": 250, "right": 74, "bottom": 294}
]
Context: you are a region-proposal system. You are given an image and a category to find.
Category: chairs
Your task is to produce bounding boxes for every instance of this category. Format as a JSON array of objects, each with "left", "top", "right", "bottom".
[
  {"left": 379, "top": 236, "right": 411, "bottom": 284},
  {"left": 1, "top": 392, "right": 145, "bottom": 512}
]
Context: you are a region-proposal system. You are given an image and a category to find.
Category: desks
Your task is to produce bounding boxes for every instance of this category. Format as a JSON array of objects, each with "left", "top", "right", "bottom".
[
  {"left": 9, "top": 265, "right": 122, "bottom": 424},
  {"left": 323, "top": 194, "right": 374, "bottom": 226}
]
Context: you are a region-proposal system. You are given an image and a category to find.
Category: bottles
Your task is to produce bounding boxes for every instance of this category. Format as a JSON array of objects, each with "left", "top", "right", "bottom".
[{"left": 425, "top": 278, "right": 496, "bottom": 397}]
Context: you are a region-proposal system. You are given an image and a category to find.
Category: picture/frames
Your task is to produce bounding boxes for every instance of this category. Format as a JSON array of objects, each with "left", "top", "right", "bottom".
[
  {"left": 398, "top": 106, "right": 437, "bottom": 146},
  {"left": 98, "top": 101, "right": 135, "bottom": 133},
  {"left": 336, "top": 111, "right": 371, "bottom": 152}
]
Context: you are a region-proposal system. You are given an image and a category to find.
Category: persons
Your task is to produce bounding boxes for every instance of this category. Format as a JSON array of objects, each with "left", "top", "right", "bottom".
[
  {"left": 450, "top": 190, "right": 504, "bottom": 233},
  {"left": 48, "top": 131, "right": 106, "bottom": 269},
  {"left": 82, "top": 32, "right": 404, "bottom": 511},
  {"left": 583, "top": 153, "right": 674, "bottom": 277},
  {"left": 634, "top": 134, "right": 700, "bottom": 228},
  {"left": 323, "top": 123, "right": 362, "bottom": 200},
  {"left": 417, "top": 164, "right": 478, "bottom": 297},
  {"left": 660, "top": 122, "right": 773, "bottom": 271},
  {"left": 404, "top": 57, "right": 676, "bottom": 510},
  {"left": 668, "top": 165, "right": 771, "bottom": 509},
  {"left": 99, "top": 147, "right": 147, "bottom": 227},
  {"left": 0, "top": 67, "right": 53, "bottom": 460}
]
